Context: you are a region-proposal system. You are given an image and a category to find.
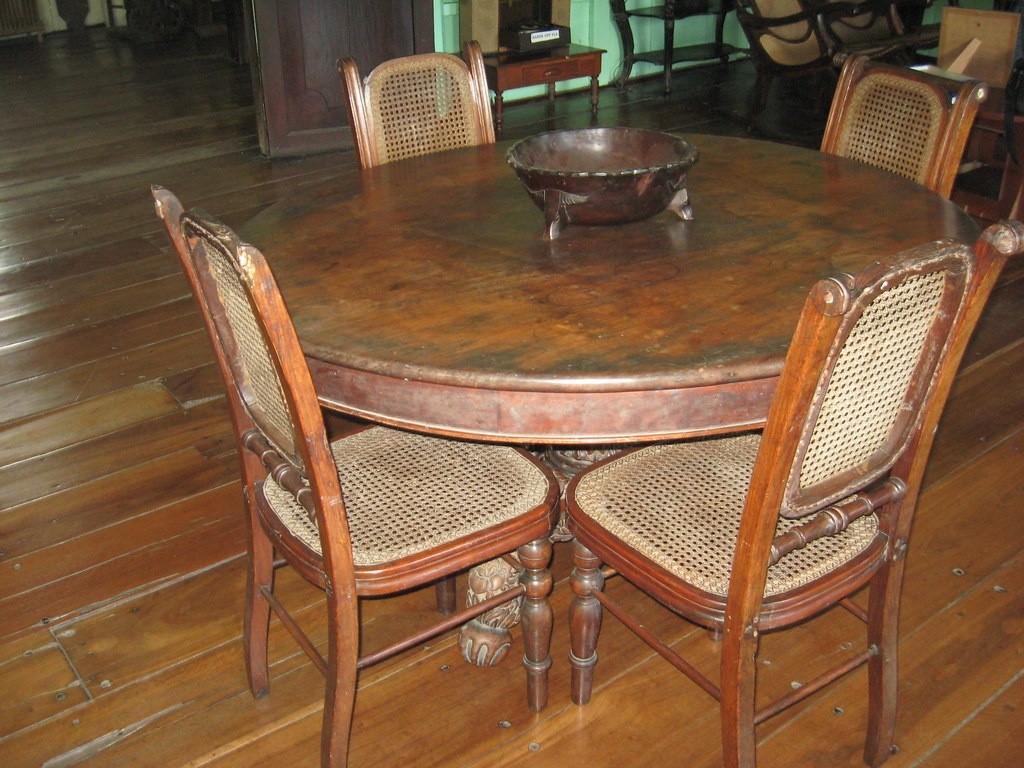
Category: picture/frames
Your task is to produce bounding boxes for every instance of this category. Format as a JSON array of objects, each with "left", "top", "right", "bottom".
[{"left": 938, "top": 5, "right": 1021, "bottom": 90}]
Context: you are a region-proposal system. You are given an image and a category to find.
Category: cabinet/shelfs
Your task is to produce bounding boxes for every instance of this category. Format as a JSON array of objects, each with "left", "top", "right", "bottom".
[{"left": 610, "top": 0, "right": 752, "bottom": 91}]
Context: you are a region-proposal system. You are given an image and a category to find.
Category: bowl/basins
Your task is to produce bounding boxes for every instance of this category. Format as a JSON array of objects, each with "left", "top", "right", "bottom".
[{"left": 505, "top": 126, "right": 701, "bottom": 240}]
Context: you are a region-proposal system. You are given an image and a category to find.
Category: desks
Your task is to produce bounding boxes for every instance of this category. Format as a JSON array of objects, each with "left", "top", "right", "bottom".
[
  {"left": 242, "top": 136, "right": 977, "bottom": 669},
  {"left": 481, "top": 45, "right": 607, "bottom": 130}
]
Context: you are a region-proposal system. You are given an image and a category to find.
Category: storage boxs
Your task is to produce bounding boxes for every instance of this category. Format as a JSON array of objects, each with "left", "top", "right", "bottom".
[{"left": 501, "top": 22, "right": 571, "bottom": 51}]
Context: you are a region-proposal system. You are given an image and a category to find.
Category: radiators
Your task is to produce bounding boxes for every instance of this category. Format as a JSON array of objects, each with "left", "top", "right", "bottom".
[{"left": 0, "top": 0, "right": 46, "bottom": 40}]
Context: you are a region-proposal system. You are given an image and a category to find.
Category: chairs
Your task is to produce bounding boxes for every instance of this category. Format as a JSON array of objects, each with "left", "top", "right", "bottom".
[
  {"left": 564, "top": 215, "right": 1024, "bottom": 768},
  {"left": 148, "top": 183, "right": 551, "bottom": 768},
  {"left": 335, "top": 40, "right": 496, "bottom": 169},
  {"left": 732, "top": 0, "right": 988, "bottom": 199}
]
